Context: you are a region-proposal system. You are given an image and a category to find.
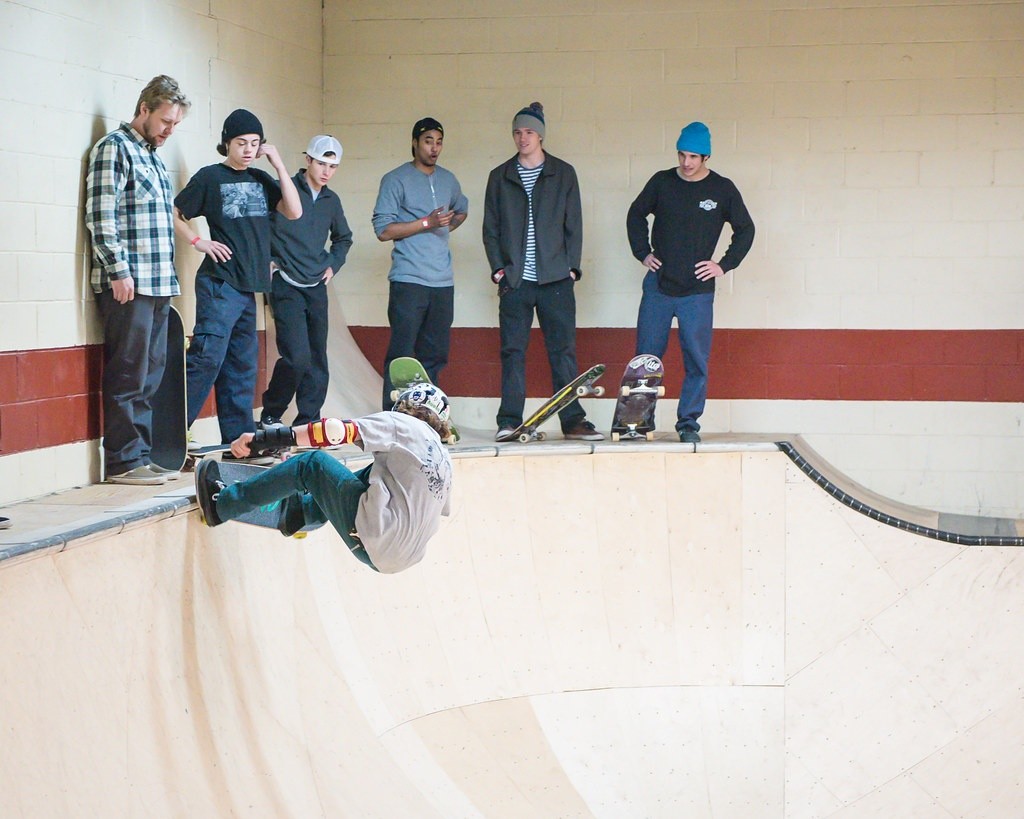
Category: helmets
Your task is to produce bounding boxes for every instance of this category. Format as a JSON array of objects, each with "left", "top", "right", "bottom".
[{"left": 392, "top": 383, "right": 451, "bottom": 424}]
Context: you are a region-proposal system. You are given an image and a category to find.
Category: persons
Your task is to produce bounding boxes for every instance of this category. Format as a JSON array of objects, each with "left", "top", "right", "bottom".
[
  {"left": 173, "top": 109, "right": 303, "bottom": 466},
  {"left": 193, "top": 382, "right": 453, "bottom": 574},
  {"left": 260, "top": 135, "right": 353, "bottom": 428},
  {"left": 482, "top": 102, "right": 605, "bottom": 442},
  {"left": 627, "top": 121, "right": 756, "bottom": 442},
  {"left": 370, "top": 117, "right": 468, "bottom": 411},
  {"left": 85, "top": 74, "right": 181, "bottom": 485}
]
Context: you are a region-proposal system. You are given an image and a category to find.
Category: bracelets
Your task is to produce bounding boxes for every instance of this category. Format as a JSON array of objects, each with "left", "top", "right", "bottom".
[
  {"left": 422, "top": 216, "right": 431, "bottom": 230},
  {"left": 189, "top": 237, "right": 200, "bottom": 246},
  {"left": 494, "top": 270, "right": 504, "bottom": 281}
]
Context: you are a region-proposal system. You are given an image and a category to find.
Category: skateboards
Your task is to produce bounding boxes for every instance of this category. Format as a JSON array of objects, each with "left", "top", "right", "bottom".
[
  {"left": 188, "top": 436, "right": 295, "bottom": 467},
  {"left": 610, "top": 353, "right": 665, "bottom": 444},
  {"left": 146, "top": 302, "right": 187, "bottom": 471},
  {"left": 195, "top": 461, "right": 332, "bottom": 539},
  {"left": 388, "top": 357, "right": 461, "bottom": 445},
  {"left": 496, "top": 364, "right": 606, "bottom": 443}
]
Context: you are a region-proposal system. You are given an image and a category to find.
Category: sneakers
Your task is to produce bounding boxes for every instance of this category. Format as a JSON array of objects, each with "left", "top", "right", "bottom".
[
  {"left": 200, "top": 458, "right": 227, "bottom": 527},
  {"left": 565, "top": 421, "right": 603, "bottom": 440},
  {"left": 220, "top": 450, "right": 274, "bottom": 465},
  {"left": 147, "top": 462, "right": 179, "bottom": 480},
  {"left": 260, "top": 414, "right": 284, "bottom": 429},
  {"left": 107, "top": 466, "right": 167, "bottom": 485},
  {"left": 679, "top": 426, "right": 701, "bottom": 442},
  {"left": 280, "top": 492, "right": 305, "bottom": 538},
  {"left": 495, "top": 424, "right": 514, "bottom": 438}
]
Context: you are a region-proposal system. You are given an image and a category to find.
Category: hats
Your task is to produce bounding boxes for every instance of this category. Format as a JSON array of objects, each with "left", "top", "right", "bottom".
[
  {"left": 222, "top": 109, "right": 263, "bottom": 141},
  {"left": 512, "top": 102, "right": 545, "bottom": 138},
  {"left": 303, "top": 134, "right": 343, "bottom": 165},
  {"left": 412, "top": 119, "right": 443, "bottom": 157},
  {"left": 677, "top": 122, "right": 710, "bottom": 156}
]
